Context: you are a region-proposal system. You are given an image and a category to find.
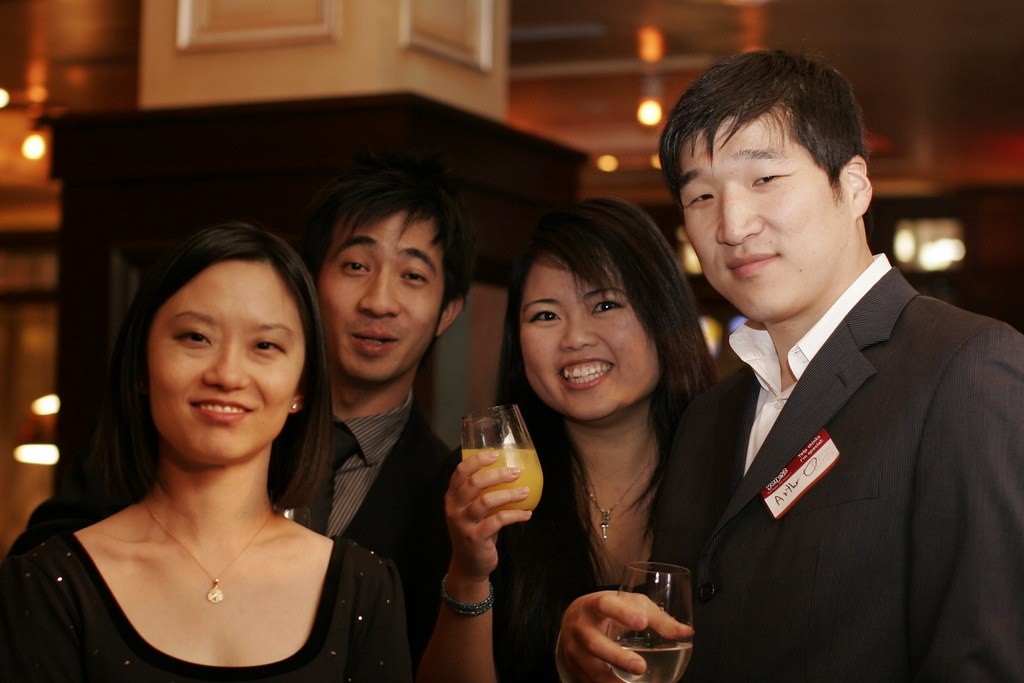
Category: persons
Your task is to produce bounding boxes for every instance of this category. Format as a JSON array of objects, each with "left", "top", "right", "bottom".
[
  {"left": 0, "top": 222, "right": 410, "bottom": 683},
  {"left": 268, "top": 163, "right": 479, "bottom": 683},
  {"left": 555, "top": 47, "right": 1024, "bottom": 683},
  {"left": 417, "top": 192, "right": 715, "bottom": 683}
]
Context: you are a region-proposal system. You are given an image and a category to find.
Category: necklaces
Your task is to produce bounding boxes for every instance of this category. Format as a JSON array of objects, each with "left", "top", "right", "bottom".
[
  {"left": 140, "top": 498, "right": 274, "bottom": 602},
  {"left": 569, "top": 450, "right": 652, "bottom": 541}
]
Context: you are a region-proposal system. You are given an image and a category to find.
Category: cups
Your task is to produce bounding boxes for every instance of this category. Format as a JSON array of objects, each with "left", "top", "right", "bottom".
[
  {"left": 608, "top": 562, "right": 695, "bottom": 683},
  {"left": 460, "top": 403, "right": 544, "bottom": 518}
]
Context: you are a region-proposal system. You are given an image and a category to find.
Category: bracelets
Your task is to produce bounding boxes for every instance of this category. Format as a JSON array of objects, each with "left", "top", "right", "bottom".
[{"left": 438, "top": 573, "right": 496, "bottom": 617}]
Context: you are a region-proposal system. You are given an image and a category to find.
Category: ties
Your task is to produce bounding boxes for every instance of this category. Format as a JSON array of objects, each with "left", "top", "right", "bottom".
[{"left": 315, "top": 424, "right": 363, "bottom": 536}]
